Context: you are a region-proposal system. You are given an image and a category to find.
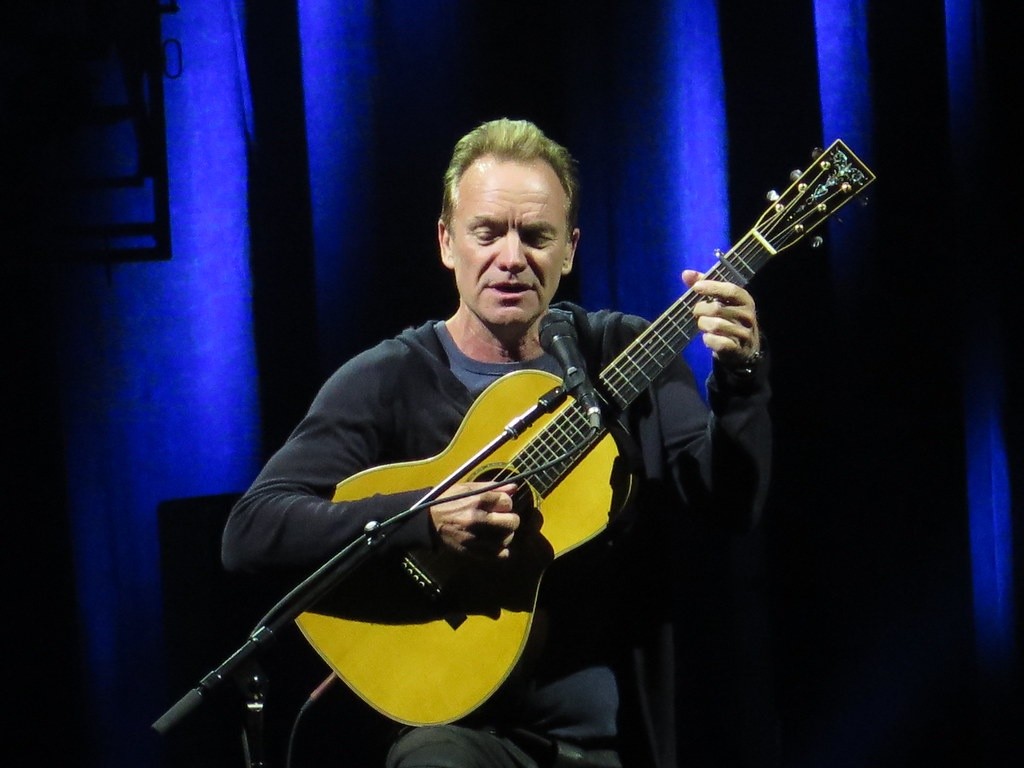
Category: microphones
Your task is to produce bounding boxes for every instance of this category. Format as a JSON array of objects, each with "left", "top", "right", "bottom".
[{"left": 538, "top": 312, "right": 601, "bottom": 432}]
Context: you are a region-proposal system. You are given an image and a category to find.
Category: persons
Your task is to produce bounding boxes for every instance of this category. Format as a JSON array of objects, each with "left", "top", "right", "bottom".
[{"left": 220, "top": 117, "right": 774, "bottom": 768}]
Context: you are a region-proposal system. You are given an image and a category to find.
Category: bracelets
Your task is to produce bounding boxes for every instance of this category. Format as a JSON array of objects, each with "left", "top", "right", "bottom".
[{"left": 710, "top": 347, "right": 768, "bottom": 384}]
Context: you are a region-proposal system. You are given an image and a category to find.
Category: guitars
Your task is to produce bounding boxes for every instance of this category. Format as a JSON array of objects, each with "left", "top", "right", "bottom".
[{"left": 292, "top": 139, "right": 876, "bottom": 728}]
